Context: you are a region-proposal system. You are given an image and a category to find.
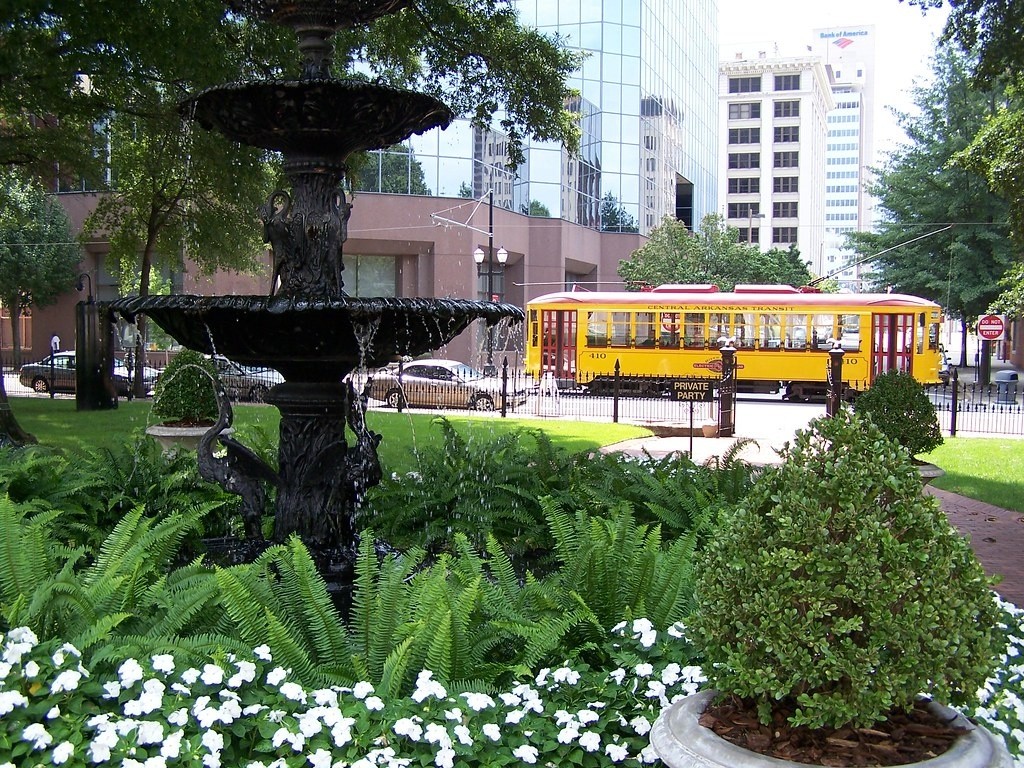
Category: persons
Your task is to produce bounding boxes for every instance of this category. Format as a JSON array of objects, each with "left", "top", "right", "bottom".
[{"left": 50, "top": 331, "right": 61, "bottom": 354}]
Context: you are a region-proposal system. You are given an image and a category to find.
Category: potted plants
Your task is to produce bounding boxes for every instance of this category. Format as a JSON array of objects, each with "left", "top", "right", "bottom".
[
  {"left": 145, "top": 349, "right": 236, "bottom": 453},
  {"left": 649, "top": 399, "right": 1012, "bottom": 768},
  {"left": 852, "top": 367, "right": 944, "bottom": 489}
]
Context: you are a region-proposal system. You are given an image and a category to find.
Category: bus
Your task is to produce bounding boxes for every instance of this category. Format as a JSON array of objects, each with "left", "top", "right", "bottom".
[{"left": 510, "top": 279, "right": 951, "bottom": 405}]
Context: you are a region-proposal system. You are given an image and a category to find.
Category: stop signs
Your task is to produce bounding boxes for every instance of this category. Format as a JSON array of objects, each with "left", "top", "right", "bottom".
[
  {"left": 976, "top": 315, "right": 1006, "bottom": 341},
  {"left": 661, "top": 313, "right": 682, "bottom": 333}
]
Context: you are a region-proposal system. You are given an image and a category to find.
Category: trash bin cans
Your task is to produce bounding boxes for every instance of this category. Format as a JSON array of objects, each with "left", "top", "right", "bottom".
[{"left": 994, "top": 370, "right": 1020, "bottom": 404}]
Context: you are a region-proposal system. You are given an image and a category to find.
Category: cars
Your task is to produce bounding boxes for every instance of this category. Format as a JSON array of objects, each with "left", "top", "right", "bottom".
[
  {"left": 367, "top": 358, "right": 528, "bottom": 412},
  {"left": 18, "top": 349, "right": 158, "bottom": 398},
  {"left": 904, "top": 342, "right": 953, "bottom": 387},
  {"left": 203, "top": 355, "right": 286, "bottom": 403}
]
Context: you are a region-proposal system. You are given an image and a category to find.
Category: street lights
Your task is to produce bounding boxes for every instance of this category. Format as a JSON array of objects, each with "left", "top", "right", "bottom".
[
  {"left": 472, "top": 193, "right": 511, "bottom": 379},
  {"left": 748, "top": 209, "right": 766, "bottom": 248}
]
